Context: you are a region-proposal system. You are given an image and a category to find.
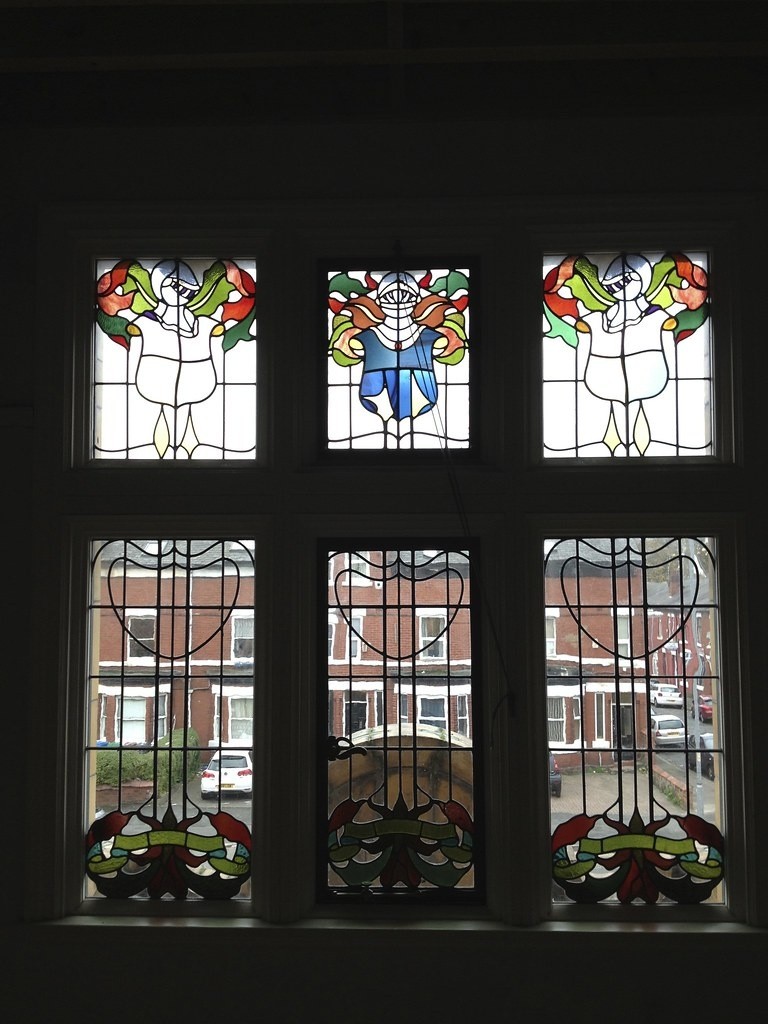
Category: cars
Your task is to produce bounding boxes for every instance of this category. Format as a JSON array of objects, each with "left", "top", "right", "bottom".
[
  {"left": 689, "top": 733, "right": 714, "bottom": 781},
  {"left": 691, "top": 695, "right": 712, "bottom": 723},
  {"left": 651, "top": 714, "right": 685, "bottom": 748},
  {"left": 201, "top": 749, "right": 252, "bottom": 800},
  {"left": 650, "top": 680, "right": 683, "bottom": 709},
  {"left": 549, "top": 751, "right": 561, "bottom": 797}
]
